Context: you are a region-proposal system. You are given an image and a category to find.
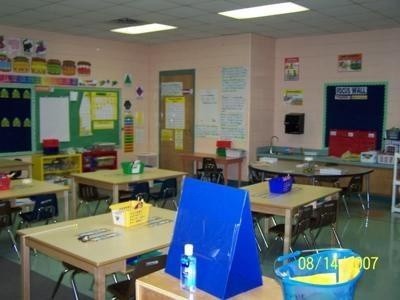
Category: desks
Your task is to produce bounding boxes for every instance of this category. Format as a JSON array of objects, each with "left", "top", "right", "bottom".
[
  {"left": 71, "top": 166, "right": 190, "bottom": 218},
  {"left": 1, "top": 178, "right": 70, "bottom": 221},
  {"left": 180, "top": 151, "right": 244, "bottom": 188},
  {"left": 247, "top": 160, "right": 374, "bottom": 218},
  {"left": 240, "top": 181, "right": 343, "bottom": 264},
  {"left": 15, "top": 206, "right": 177, "bottom": 300}
]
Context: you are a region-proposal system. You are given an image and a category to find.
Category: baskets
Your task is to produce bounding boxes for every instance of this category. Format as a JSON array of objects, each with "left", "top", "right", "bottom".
[
  {"left": 377, "top": 129, "right": 400, "bottom": 164},
  {"left": 120, "top": 161, "right": 144, "bottom": 174},
  {"left": 0, "top": 173, "right": 10, "bottom": 190},
  {"left": 268, "top": 175, "right": 293, "bottom": 194},
  {"left": 109, "top": 200, "right": 153, "bottom": 228}
]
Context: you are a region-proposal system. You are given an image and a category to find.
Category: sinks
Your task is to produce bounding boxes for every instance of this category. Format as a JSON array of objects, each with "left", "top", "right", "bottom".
[{"left": 271, "top": 152, "right": 292, "bottom": 156}]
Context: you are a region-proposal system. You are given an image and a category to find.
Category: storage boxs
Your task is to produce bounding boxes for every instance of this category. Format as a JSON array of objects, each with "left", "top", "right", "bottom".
[{"left": 135, "top": 268, "right": 283, "bottom": 298}]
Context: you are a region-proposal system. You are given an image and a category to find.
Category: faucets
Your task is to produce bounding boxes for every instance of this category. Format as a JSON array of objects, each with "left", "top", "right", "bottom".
[{"left": 269, "top": 136, "right": 279, "bottom": 154}]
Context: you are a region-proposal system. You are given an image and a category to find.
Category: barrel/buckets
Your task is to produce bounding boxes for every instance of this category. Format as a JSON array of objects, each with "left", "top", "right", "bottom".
[{"left": 274, "top": 247, "right": 365, "bottom": 300}]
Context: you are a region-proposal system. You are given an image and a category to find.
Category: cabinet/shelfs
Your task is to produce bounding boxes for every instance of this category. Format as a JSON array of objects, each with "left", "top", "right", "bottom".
[{"left": 29, "top": 151, "right": 117, "bottom": 182}]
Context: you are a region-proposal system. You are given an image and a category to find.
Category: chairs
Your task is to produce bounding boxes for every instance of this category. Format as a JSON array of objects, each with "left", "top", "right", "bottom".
[
  {"left": 49, "top": 261, "right": 118, "bottom": 299},
  {"left": 264, "top": 205, "right": 314, "bottom": 258},
  {"left": 105, "top": 255, "right": 166, "bottom": 298},
  {"left": 76, "top": 184, "right": 110, "bottom": 217},
  {"left": 120, "top": 182, "right": 151, "bottom": 204},
  {"left": 305, "top": 199, "right": 343, "bottom": 248},
  {"left": 14, "top": 192, "right": 59, "bottom": 257},
  {"left": 151, "top": 176, "right": 177, "bottom": 210},
  {"left": 252, "top": 211, "right": 269, "bottom": 252},
  {"left": 1, "top": 199, "right": 20, "bottom": 257}
]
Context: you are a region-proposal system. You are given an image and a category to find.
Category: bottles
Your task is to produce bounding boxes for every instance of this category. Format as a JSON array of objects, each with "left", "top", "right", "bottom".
[{"left": 179, "top": 243, "right": 196, "bottom": 293}]
